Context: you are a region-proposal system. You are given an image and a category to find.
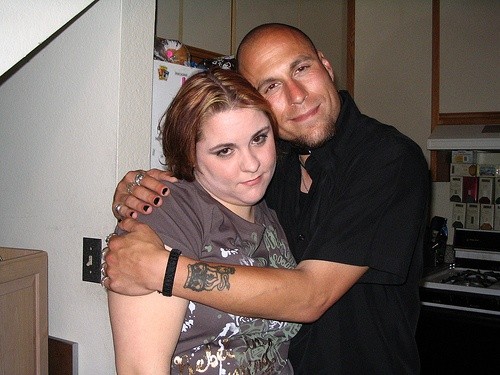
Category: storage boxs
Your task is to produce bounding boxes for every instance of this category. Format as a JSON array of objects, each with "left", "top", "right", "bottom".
[{"left": 448, "top": 150, "right": 500, "bottom": 231}]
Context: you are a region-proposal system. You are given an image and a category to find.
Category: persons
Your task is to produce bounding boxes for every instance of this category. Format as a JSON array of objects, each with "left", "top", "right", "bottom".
[
  {"left": 107, "top": 66, "right": 304, "bottom": 375},
  {"left": 99, "top": 23, "right": 431, "bottom": 375}
]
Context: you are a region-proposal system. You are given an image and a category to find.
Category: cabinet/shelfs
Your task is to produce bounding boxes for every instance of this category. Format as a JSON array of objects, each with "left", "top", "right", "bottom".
[
  {"left": 348, "top": 1, "right": 440, "bottom": 175},
  {"left": 433, "top": 0, "right": 500, "bottom": 126},
  {"left": 155, "top": 1, "right": 231, "bottom": 61},
  {"left": 230, "top": 0, "right": 351, "bottom": 95}
]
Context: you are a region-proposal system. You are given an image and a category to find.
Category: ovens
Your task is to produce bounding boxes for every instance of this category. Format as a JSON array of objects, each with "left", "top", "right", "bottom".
[{"left": 416, "top": 306, "right": 500, "bottom": 375}]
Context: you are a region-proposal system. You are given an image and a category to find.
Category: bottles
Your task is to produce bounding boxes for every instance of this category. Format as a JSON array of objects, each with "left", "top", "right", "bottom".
[{"left": 444, "top": 245, "right": 456, "bottom": 264}]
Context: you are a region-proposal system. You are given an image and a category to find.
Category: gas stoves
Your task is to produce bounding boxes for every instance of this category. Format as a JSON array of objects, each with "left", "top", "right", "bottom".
[{"left": 419, "top": 227, "right": 500, "bottom": 311}]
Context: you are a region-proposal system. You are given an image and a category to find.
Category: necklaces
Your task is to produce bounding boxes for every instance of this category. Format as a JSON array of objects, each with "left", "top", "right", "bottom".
[{"left": 298, "top": 155, "right": 305, "bottom": 170}]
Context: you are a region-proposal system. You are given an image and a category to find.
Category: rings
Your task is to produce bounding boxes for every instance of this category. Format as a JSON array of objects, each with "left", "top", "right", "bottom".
[
  {"left": 126, "top": 183, "right": 136, "bottom": 193},
  {"left": 135, "top": 173, "right": 146, "bottom": 185},
  {"left": 101, "top": 233, "right": 118, "bottom": 288},
  {"left": 115, "top": 204, "right": 122, "bottom": 213}
]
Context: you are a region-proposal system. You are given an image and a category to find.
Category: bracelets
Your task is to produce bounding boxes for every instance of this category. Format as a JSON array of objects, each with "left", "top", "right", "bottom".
[{"left": 162, "top": 249, "right": 182, "bottom": 297}]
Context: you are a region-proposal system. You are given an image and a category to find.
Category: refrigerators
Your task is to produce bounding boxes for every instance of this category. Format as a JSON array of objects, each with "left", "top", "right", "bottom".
[{"left": 148, "top": 59, "right": 204, "bottom": 175}]
